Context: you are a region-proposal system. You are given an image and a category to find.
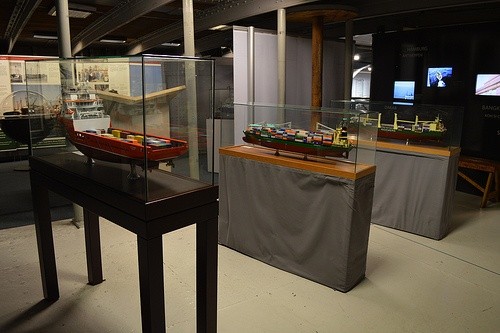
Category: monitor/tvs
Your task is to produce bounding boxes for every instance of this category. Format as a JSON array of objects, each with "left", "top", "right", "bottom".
[
  {"left": 475, "top": 74, "right": 500, "bottom": 96},
  {"left": 427, "top": 67, "right": 453, "bottom": 88},
  {"left": 392, "top": 81, "right": 415, "bottom": 102}
]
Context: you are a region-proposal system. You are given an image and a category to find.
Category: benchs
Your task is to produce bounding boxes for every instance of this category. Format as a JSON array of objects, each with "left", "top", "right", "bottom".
[{"left": 456, "top": 155, "right": 500, "bottom": 210}]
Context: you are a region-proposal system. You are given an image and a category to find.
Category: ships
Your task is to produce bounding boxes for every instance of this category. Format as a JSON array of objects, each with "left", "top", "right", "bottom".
[
  {"left": 241, "top": 121, "right": 354, "bottom": 159},
  {"left": 341, "top": 113, "right": 449, "bottom": 145},
  {"left": 57, "top": 88, "right": 188, "bottom": 170}
]
